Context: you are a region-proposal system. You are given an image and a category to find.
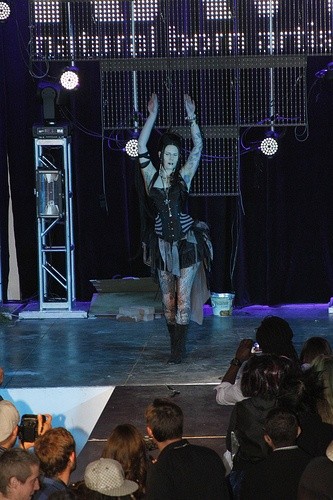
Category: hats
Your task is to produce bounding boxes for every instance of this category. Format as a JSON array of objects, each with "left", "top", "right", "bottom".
[
  {"left": 0, "top": 400, "right": 19, "bottom": 441},
  {"left": 83, "top": 458, "right": 137, "bottom": 496}
]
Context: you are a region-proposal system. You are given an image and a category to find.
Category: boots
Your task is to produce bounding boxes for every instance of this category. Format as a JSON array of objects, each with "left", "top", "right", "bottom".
[
  {"left": 174, "top": 323, "right": 188, "bottom": 364},
  {"left": 167, "top": 324, "right": 175, "bottom": 364}
]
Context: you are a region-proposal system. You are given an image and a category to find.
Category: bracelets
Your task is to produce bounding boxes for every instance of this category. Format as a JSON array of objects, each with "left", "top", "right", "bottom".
[
  {"left": 188, "top": 120, "right": 198, "bottom": 127},
  {"left": 185, "top": 114, "right": 196, "bottom": 119}
]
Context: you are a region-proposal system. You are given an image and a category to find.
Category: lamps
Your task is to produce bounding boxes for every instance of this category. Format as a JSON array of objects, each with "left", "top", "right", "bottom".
[
  {"left": 122, "top": 130, "right": 140, "bottom": 162},
  {"left": 58, "top": 65, "right": 80, "bottom": 92},
  {"left": 0, "top": 0, "right": 15, "bottom": 24},
  {"left": 257, "top": 130, "right": 280, "bottom": 160}
]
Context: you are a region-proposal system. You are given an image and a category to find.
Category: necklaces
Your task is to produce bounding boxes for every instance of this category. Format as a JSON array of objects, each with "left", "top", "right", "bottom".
[{"left": 160, "top": 170, "right": 174, "bottom": 217}]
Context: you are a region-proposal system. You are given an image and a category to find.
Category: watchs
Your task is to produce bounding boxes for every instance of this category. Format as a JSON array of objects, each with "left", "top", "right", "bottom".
[{"left": 230, "top": 358, "right": 243, "bottom": 368}]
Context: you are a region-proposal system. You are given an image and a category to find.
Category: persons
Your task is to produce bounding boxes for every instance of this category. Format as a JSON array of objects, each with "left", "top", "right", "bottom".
[
  {"left": 137, "top": 93, "right": 214, "bottom": 364},
  {"left": 230, "top": 407, "right": 314, "bottom": 500},
  {"left": 145, "top": 398, "right": 231, "bottom": 500},
  {"left": 101, "top": 424, "right": 153, "bottom": 492},
  {"left": 0, "top": 400, "right": 53, "bottom": 457},
  {"left": 0, "top": 448, "right": 40, "bottom": 500},
  {"left": 226, "top": 337, "right": 333, "bottom": 500},
  {"left": 212, "top": 315, "right": 302, "bottom": 406},
  {"left": 30, "top": 427, "right": 77, "bottom": 500}
]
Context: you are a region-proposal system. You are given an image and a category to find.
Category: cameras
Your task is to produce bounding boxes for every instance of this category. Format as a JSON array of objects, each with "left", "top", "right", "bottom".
[
  {"left": 253, "top": 342, "right": 262, "bottom": 351},
  {"left": 18, "top": 414, "right": 46, "bottom": 442}
]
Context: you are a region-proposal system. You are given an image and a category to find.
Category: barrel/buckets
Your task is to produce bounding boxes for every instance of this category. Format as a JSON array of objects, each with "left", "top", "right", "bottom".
[{"left": 211, "top": 292, "right": 235, "bottom": 318}]
